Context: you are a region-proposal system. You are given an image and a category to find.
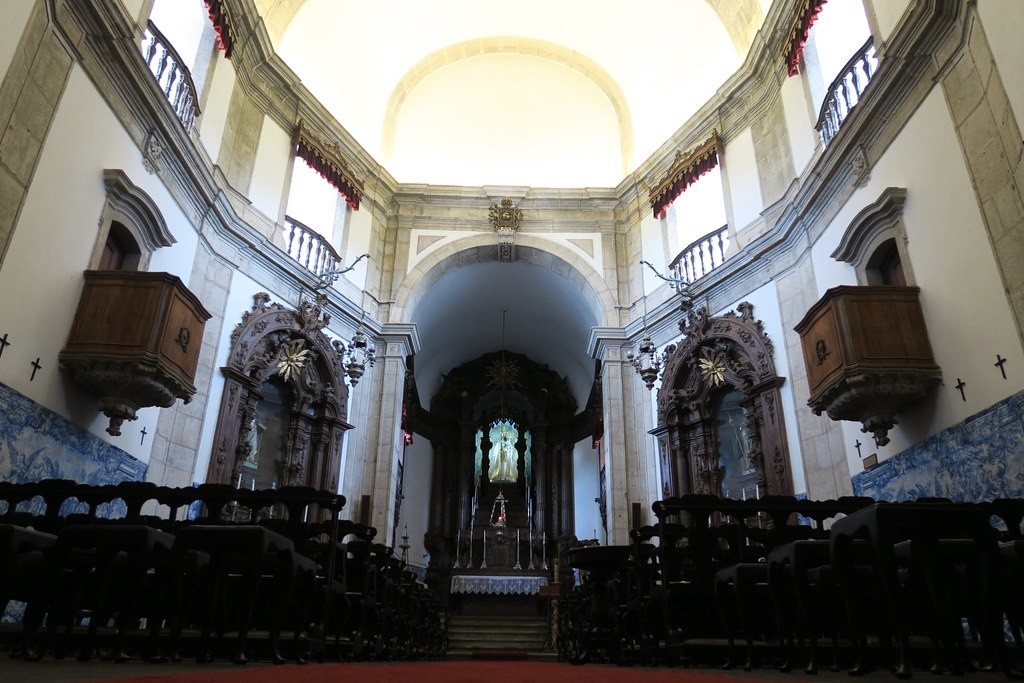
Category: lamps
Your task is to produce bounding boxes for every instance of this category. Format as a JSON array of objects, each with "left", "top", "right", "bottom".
[
  {"left": 626, "top": 259, "right": 695, "bottom": 390},
  {"left": 315, "top": 253, "right": 376, "bottom": 388},
  {"left": 488, "top": 307, "right": 508, "bottom": 542}
]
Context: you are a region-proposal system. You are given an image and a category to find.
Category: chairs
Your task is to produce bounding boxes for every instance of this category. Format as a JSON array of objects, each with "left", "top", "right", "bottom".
[
  {"left": 556, "top": 490, "right": 1024, "bottom": 682},
  {"left": 0, "top": 477, "right": 448, "bottom": 664}
]
